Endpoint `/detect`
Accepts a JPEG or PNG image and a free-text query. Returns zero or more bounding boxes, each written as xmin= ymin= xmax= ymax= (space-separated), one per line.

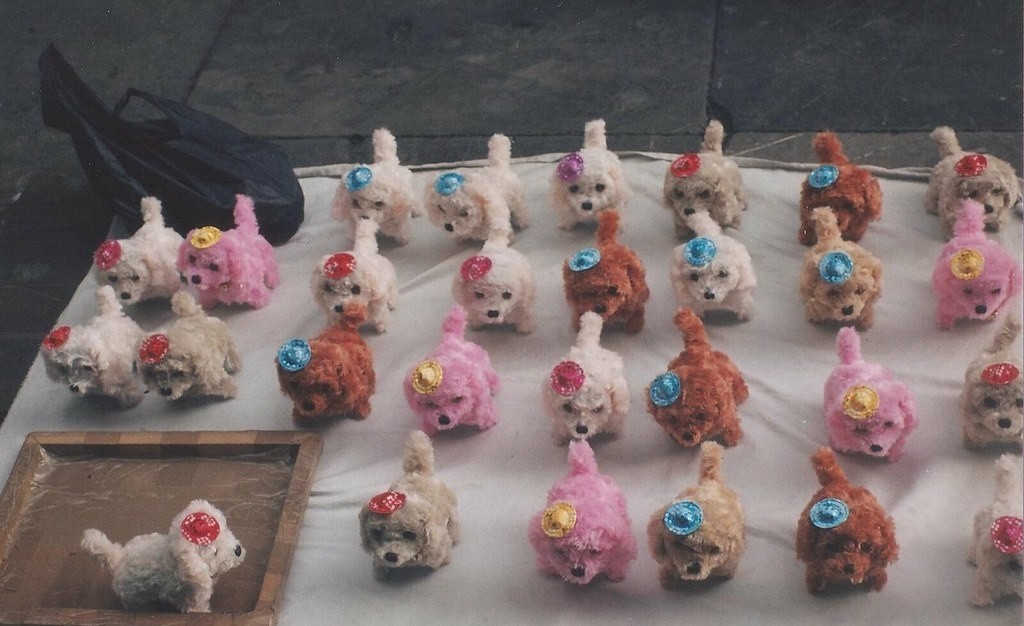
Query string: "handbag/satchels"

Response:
xmin=38 ymin=45 xmax=304 ymax=246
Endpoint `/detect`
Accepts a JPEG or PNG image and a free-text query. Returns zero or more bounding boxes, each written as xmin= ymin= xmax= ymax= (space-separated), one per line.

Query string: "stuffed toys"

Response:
xmin=358 ymin=432 xmax=460 ymax=579
xmin=40 ymin=285 xmax=148 ymax=409
xmin=968 ymin=453 xmax=1023 ymax=606
xmin=526 ymin=440 xmax=637 ymax=584
xmin=310 ymin=220 xmax=399 ymax=332
xmin=428 ymin=118 xmax=1024 ymax=333
xmin=404 ymin=306 xmax=501 ymax=436
xmin=647 ymin=440 xmax=746 ymax=591
xmin=824 ymin=326 xmax=916 ymax=457
xmin=93 ymin=196 xmax=185 ymax=306
xmin=645 ymin=307 xmax=748 ymax=446
xmin=795 ymin=449 xmax=900 ymax=592
xmin=177 ymin=194 xmax=280 ymax=310
xmin=960 ymin=312 xmax=1024 ymax=449
xmin=137 ymin=290 xmax=242 ymax=401
xmin=334 ymin=127 xmax=424 ymax=244
xmin=275 ymin=300 xmax=375 ymax=422
xmin=540 ymin=311 xmax=630 ymax=444
xmin=82 ymin=499 xmax=246 ymax=613
